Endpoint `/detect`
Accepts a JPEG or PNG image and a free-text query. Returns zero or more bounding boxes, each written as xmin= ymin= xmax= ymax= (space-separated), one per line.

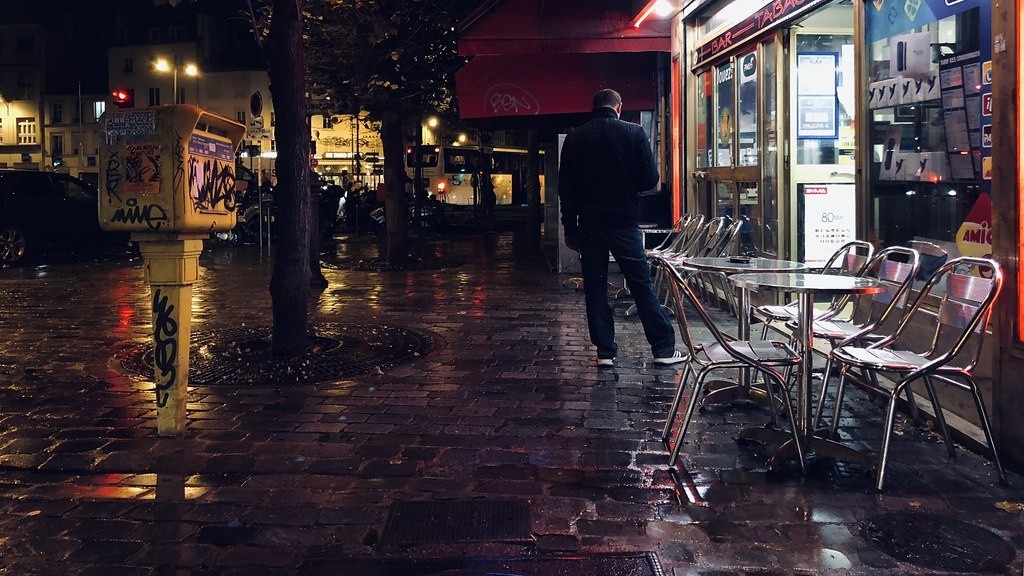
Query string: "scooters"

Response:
xmin=234 ymin=151 xmax=384 ymax=231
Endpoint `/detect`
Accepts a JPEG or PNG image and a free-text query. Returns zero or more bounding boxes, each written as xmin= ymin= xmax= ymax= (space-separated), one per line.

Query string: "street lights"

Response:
xmin=156 ymin=60 xmax=198 ymax=103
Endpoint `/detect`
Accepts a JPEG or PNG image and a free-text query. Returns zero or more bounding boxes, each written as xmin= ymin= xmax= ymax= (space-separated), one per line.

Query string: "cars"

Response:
xmin=0 ymin=169 xmax=98 ymax=229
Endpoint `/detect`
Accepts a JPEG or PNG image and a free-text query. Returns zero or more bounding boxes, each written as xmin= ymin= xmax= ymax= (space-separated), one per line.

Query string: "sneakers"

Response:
xmin=596 ymin=355 xmax=615 ymax=365
xmin=652 ymin=348 xmax=687 ymax=364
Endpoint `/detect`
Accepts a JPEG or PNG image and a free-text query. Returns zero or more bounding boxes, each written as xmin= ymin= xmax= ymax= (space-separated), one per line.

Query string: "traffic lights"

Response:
xmin=112 ymin=89 xmax=131 ymax=105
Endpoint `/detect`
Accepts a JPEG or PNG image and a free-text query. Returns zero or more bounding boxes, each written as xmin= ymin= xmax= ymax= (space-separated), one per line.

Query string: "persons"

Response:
xmin=363 ymin=183 xmax=369 ymax=194
xmin=556 ymin=89 xmax=690 ymax=367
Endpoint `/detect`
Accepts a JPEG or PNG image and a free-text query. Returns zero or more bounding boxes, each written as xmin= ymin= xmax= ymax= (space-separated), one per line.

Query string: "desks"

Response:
xmin=683 ymin=257 xmax=809 ymax=416
xmin=615 ymin=224 xmax=679 ymax=316
xmin=727 ymin=273 xmax=888 ymax=478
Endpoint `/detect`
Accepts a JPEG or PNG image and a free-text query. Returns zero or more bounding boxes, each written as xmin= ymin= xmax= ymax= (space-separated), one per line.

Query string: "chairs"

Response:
xmin=643 ymin=213 xmax=1008 ymax=490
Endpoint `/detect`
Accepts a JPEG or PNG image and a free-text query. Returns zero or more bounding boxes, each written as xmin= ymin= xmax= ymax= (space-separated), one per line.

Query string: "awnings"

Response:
xmin=455 ymin=0 xmax=672 ymax=118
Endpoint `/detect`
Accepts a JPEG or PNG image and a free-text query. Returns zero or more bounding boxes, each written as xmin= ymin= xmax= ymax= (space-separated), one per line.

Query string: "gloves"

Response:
xmin=565 ymin=236 xmax=578 ymax=250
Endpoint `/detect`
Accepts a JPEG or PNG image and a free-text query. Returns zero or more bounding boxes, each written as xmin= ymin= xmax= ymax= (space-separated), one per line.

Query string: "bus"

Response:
xmin=404 ymin=144 xmax=546 ymax=208
